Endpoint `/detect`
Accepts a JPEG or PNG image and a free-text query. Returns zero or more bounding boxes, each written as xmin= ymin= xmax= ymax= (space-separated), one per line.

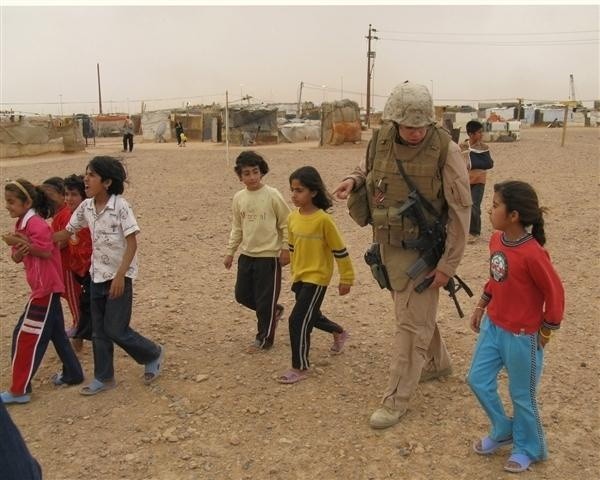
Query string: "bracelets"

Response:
xmin=474 ymin=306 xmax=486 ymax=312
xmin=538 ymin=327 xmax=554 ymax=339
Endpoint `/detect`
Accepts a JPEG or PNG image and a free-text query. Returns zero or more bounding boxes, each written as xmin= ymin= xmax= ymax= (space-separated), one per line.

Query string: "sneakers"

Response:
xmin=420 ymin=367 xmax=453 ymax=382
xmin=370 ymin=405 xmax=404 ymax=429
xmin=273 ymin=304 xmax=285 ymax=328
xmin=246 ymin=345 xmax=264 ymax=354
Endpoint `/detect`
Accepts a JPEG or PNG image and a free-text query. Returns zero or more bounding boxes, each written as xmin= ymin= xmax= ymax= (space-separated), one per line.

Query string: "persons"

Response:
xmin=276 ymin=165 xmax=355 ymax=385
xmin=178 ymin=131 xmax=186 ymax=147
xmin=51 ymin=156 xmax=165 ymax=396
xmin=1 ymin=177 xmax=84 ymax=402
xmin=464 ymin=180 xmax=566 ymax=473
xmin=330 ymin=81 xmax=473 ymax=429
xmin=458 ymin=120 xmax=494 ymax=244
xmin=43 ymin=175 xmax=92 ymax=337
xmin=120 ymin=114 xmax=135 ymax=153
xmin=174 ymin=118 xmax=184 ymax=145
xmin=222 ymin=149 xmax=292 ymax=349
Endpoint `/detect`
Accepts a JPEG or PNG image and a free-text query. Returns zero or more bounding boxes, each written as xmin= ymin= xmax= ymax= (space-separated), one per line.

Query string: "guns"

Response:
xmin=397 ymin=196 xmax=473 ymax=318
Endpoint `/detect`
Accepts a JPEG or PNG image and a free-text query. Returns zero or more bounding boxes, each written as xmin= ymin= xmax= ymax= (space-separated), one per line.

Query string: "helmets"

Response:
xmin=380 ymin=82 xmax=437 ymax=128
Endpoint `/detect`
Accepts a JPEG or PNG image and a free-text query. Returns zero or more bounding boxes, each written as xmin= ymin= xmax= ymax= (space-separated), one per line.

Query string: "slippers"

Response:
xmin=505 ymin=453 xmax=532 ymax=473
xmin=144 ymin=344 xmax=165 ymax=384
xmin=51 ymin=373 xmax=66 ymax=385
xmin=467 ymin=233 xmax=480 ymax=244
xmin=78 ymin=380 xmax=117 ymax=396
xmin=1 ymin=390 xmax=29 ymax=403
xmin=275 ymin=368 xmax=307 ymax=383
xmin=473 ymin=435 xmax=513 ymax=454
xmin=330 ymin=330 xmax=349 ymax=354
